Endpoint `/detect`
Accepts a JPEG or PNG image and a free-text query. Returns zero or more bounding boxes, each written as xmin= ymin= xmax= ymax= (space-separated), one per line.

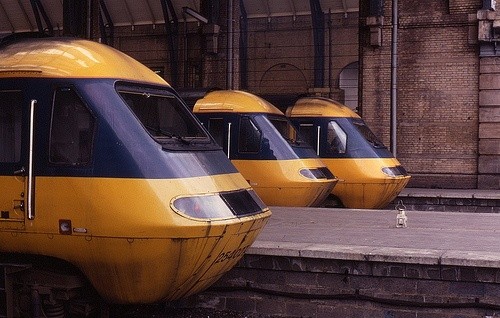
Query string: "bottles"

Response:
xmin=396 ymin=209 xmax=407 ymax=229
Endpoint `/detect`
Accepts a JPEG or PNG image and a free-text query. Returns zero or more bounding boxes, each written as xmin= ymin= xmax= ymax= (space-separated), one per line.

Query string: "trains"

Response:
xmin=258 ymin=93 xmax=413 ymax=210
xmin=177 ymin=88 xmax=337 ymax=208
xmin=0 ymin=36 xmax=274 ymax=318
